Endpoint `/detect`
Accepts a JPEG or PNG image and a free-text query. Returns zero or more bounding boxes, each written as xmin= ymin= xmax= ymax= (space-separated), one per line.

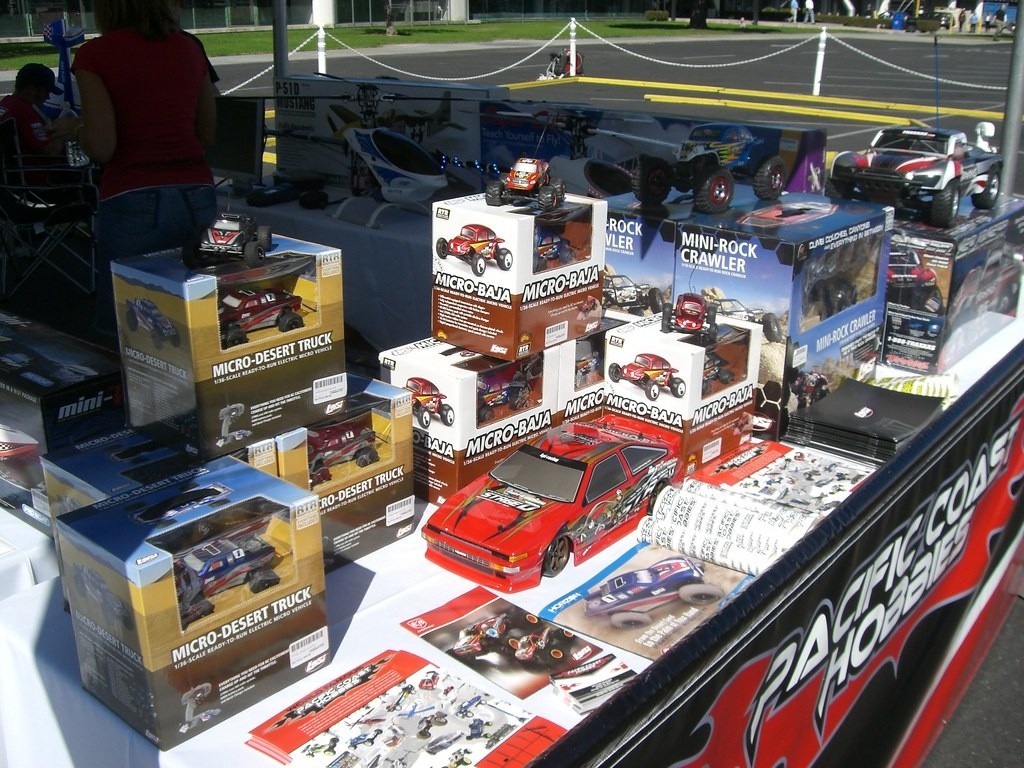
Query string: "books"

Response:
xmin=634 ymin=479 xmax=817 ymax=575
xmin=779 ymin=377 xmax=945 ymax=473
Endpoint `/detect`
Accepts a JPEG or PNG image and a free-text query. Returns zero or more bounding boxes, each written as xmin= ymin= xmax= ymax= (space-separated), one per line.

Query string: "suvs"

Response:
xmin=635 ymin=119 xmax=787 ymax=216
xmin=922 ymin=11 xmax=956 ymax=30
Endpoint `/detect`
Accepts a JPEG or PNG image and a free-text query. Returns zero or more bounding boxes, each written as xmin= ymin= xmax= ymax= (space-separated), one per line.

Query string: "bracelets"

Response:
xmin=73 ymin=122 xmax=88 ymax=139
xmin=49 ymin=123 xmax=57 ymax=132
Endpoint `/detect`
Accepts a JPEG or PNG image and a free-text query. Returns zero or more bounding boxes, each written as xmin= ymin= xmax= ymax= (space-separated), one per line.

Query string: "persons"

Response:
xmin=957 ymin=8 xmax=967 ymax=32
xmin=40 ymin=0 xmax=222 ymax=347
xmin=787 ymin=0 xmax=799 ymax=24
xmin=984 ymin=12 xmax=992 ymax=32
xmin=970 ymin=12 xmax=979 ymax=33
xmin=865 ymin=4 xmax=873 ymax=19
xmin=991 ymin=3 xmax=1007 ymax=42
xmin=804 ymin=0 xmax=815 ymax=24
xmin=0 ymin=63 xmax=99 ymax=247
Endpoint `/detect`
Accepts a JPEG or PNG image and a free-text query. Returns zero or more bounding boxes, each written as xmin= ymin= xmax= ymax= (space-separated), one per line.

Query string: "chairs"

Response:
xmin=0 ymin=116 xmax=101 ymax=300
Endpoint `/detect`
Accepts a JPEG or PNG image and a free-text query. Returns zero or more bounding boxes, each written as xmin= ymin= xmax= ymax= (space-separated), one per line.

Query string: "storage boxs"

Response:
xmin=0 ymin=74 xmax=1024 ymax=751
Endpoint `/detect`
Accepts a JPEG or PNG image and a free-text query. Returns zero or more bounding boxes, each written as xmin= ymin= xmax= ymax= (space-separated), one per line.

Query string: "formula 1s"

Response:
xmin=126 ymin=296 xmax=181 ymax=350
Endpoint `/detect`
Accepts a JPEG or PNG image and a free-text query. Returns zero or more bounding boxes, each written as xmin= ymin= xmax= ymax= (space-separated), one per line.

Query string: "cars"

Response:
xmin=438 ymin=223 xmax=516 ymax=274
xmin=830 ymin=127 xmax=1005 ymax=228
xmin=305 ymin=422 xmax=382 ymax=489
xmin=183 ymin=208 xmax=275 ymax=268
xmin=173 ymin=535 xmax=282 ymax=629
xmin=663 ymin=293 xmax=722 ymax=344
xmin=486 ymin=157 xmax=567 ymax=216
xmin=222 ymin=285 xmax=306 ymax=343
xmin=303 ymin=671 xmax=509 ymax=768
xmin=609 ymin=354 xmax=687 ymax=401
xmin=447 ymin=607 xmax=577 ymax=672
xmin=886 ymin=243 xmax=944 ymax=313
xmin=417 ymin=417 xmax=683 ymax=594
xmin=583 ymin=557 xmax=723 ymax=628
xmin=405 ymin=378 xmax=455 ymax=425
xmin=535 ymin=230 xmax=579 ymax=275
